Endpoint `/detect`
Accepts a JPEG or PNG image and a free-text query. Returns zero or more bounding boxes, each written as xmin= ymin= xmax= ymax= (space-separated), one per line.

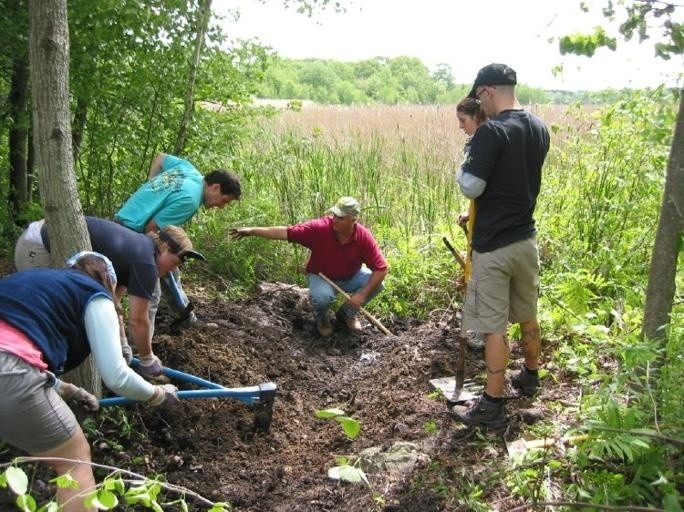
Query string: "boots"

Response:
xmin=453 ymin=391 xmax=506 ymax=424
xmin=511 ymin=366 xmax=539 ymax=395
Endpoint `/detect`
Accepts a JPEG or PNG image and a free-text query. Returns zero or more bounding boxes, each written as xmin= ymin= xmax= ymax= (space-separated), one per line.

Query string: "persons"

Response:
xmin=13 ymin=216 xmax=192 ymax=377
xmin=452 ymin=63 xmax=550 ymax=426
xmin=0 ymin=251 xmax=181 ymax=512
xmin=114 ymin=153 xmax=241 ymax=332
xmin=229 ymin=196 xmax=390 ymax=337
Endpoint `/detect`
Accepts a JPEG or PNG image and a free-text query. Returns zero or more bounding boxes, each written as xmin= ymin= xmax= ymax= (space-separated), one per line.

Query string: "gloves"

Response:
xmin=61 ymin=383 xmax=99 ymax=412
xmin=121 ymin=337 xmax=180 ymax=407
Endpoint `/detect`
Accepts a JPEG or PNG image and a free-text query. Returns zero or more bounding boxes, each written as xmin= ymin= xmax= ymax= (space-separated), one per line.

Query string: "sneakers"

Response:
xmin=317 ymin=308 xmax=362 ymax=336
xmin=172 ymin=312 xmax=218 ymax=337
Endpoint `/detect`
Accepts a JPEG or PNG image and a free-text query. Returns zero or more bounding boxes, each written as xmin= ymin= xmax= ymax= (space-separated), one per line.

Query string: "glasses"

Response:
xmin=475 ymin=84 xmax=496 ymax=105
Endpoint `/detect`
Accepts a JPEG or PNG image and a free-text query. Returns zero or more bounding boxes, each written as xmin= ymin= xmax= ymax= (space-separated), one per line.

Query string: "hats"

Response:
xmin=325 ymin=196 xmax=360 ymax=217
xmin=467 ymin=64 xmax=516 ymax=98
